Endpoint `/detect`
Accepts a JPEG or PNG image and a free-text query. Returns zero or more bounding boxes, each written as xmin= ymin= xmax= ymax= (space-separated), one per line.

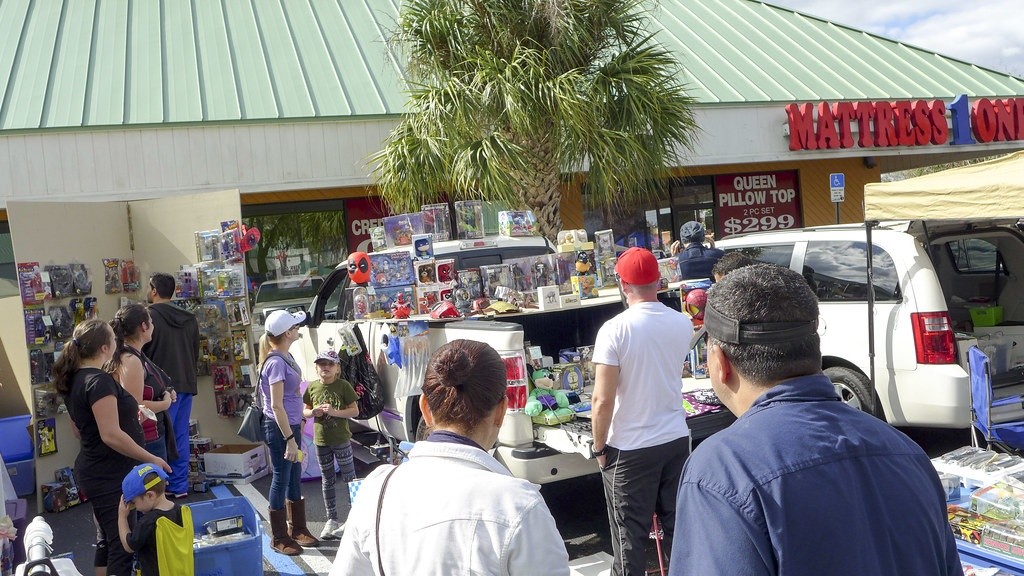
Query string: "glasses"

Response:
xmin=615 ymin=272 xmax=620 ymax=281
xmin=281 ymin=323 xmax=300 ymax=335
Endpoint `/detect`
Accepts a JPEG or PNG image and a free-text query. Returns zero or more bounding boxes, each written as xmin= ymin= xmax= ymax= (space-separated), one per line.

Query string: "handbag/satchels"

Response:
xmin=237 ymin=403 xmax=263 ymax=442
xmin=338 ymin=323 xmax=385 ymax=420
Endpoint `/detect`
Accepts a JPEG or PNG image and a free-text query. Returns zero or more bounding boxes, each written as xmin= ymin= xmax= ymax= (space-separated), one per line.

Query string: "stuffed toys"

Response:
xmin=416 ymin=239 xmax=431 ymax=256
xmin=524 ymin=369 xmax=573 ymax=416
xmin=420 ymin=266 xmax=433 ymax=283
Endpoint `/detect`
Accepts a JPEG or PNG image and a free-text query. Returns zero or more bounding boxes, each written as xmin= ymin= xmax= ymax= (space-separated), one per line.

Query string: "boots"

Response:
xmin=286 ymin=495 xmax=319 ymax=547
xmin=268 ymin=504 xmax=304 ymax=554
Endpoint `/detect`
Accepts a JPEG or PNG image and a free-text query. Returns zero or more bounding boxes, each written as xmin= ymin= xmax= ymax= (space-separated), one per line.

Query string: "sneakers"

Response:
xmin=332 ymin=523 xmax=346 ymax=538
xmin=320 ymin=519 xmax=339 ymax=539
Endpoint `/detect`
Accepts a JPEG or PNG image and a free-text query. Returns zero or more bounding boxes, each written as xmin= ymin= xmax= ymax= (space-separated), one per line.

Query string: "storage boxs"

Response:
xmin=0 ymin=415 xmax=33 ymax=461
xmin=528 ymin=346 xmax=542 ymax=360
xmin=4 ymin=458 xmax=36 ymax=543
xmin=186 ymin=366 xmax=265 ymax=476
xmin=952 ymin=306 xmax=1024 ymax=375
xmin=181 ymin=495 xmax=263 ymax=576
xmin=541 ymin=356 xmax=554 ymax=368
xmin=363 ymin=200 xmax=618 ymax=320
xmin=42 ymin=466 xmax=84 ymax=513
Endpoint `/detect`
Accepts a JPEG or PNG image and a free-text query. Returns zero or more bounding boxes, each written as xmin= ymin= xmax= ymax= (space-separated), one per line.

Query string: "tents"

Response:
xmin=864 ymin=150 xmax=1024 ymax=419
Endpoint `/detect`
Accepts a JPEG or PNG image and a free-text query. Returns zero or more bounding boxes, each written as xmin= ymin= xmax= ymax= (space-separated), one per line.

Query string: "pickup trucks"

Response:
xmin=251 ymin=275 xmax=327 ymax=355
xmin=288 ymin=233 xmax=737 ymax=522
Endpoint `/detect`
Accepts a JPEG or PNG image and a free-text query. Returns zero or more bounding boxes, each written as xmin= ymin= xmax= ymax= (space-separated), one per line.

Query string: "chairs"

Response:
xmin=964 ymin=346 xmax=1024 ymax=456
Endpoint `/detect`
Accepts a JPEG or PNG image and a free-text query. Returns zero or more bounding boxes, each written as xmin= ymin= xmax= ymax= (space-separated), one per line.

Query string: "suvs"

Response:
xmin=703 ymin=222 xmax=1024 ymax=429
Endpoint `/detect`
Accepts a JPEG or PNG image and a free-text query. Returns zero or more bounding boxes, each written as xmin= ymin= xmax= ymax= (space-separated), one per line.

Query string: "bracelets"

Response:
xmin=592 ymin=442 xmax=608 ymax=456
xmin=283 ymin=433 xmax=295 ymax=441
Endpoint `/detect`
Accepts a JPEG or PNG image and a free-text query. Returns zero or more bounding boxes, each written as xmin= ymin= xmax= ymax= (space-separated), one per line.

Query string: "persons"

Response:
xmin=713 ymin=252 xmax=753 ymax=281
xmin=671 ymin=221 xmax=725 ymax=283
xmin=353 ymin=258 xmax=414 ymax=320
xmin=103 ymin=305 xmax=176 ymax=519
xmin=591 ymin=248 xmax=694 ymax=576
xmin=258 ymin=310 xmax=319 ymax=553
xmin=53 ymin=319 xmax=172 ymax=576
xmin=118 ymin=463 xmax=194 ymax=576
xmin=303 ymin=351 xmax=359 ymax=540
xmin=142 ymin=272 xmax=199 ymax=498
xmin=669 ymin=262 xmax=964 ymax=576
xmin=329 ymin=339 xmax=571 ymax=576
xmin=513 ymin=255 xmax=575 ymax=291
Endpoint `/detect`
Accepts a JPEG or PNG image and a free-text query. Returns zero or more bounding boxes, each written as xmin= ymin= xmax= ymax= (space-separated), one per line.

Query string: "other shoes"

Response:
xmin=164 ymin=479 xmax=169 ymax=486
xmin=165 ymin=490 xmax=188 ymax=499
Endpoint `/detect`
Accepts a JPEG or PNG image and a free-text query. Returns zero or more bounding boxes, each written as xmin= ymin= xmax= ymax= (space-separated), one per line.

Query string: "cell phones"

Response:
xmin=297 ymin=450 xmax=303 ymax=462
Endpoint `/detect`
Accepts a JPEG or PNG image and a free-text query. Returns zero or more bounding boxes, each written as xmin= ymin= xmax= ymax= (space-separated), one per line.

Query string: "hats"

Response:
xmin=679 ymin=221 xmax=705 ymax=242
xmin=122 ymin=463 xmax=169 ymax=510
xmin=314 ymin=351 xmax=341 ymax=364
xmin=265 ymin=309 xmax=306 ymax=337
xmin=690 ymin=299 xmax=819 ymax=350
xmin=615 ymin=247 xmax=658 ymax=284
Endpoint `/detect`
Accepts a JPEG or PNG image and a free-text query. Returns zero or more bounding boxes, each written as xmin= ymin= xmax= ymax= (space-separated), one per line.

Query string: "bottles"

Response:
xmin=204 ymin=479 xmax=226 ymax=487
